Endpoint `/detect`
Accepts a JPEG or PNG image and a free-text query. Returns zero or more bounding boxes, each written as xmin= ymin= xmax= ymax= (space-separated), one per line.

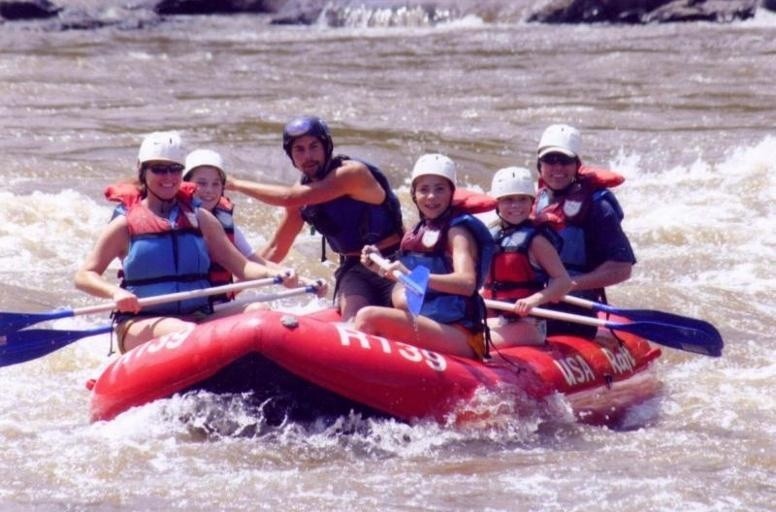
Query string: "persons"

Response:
xmin=527 ymin=124 xmax=638 ymax=340
xmin=474 ymin=165 xmax=576 ymax=351
xmin=106 ymin=147 xmax=332 ymax=305
xmin=217 ymin=112 xmax=411 ymax=322
xmin=353 ymin=150 xmax=487 ymax=362
xmin=70 ymin=127 xmax=302 ymax=356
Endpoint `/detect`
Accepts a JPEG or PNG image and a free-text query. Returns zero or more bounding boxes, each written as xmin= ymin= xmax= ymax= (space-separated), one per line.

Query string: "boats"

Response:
xmin=84 ymin=295 xmax=664 ymax=450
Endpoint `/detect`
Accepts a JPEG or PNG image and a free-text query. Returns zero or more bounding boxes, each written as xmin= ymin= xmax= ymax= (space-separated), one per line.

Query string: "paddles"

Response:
xmin=0 ymin=287 xmax=314 ymax=368
xmin=1 ymin=272 xmax=288 ymax=339
xmin=558 ymin=295 xmax=725 ymax=350
xmin=370 ymin=251 xmax=430 ymax=317
xmin=485 ymin=321 xmax=724 ymax=358
